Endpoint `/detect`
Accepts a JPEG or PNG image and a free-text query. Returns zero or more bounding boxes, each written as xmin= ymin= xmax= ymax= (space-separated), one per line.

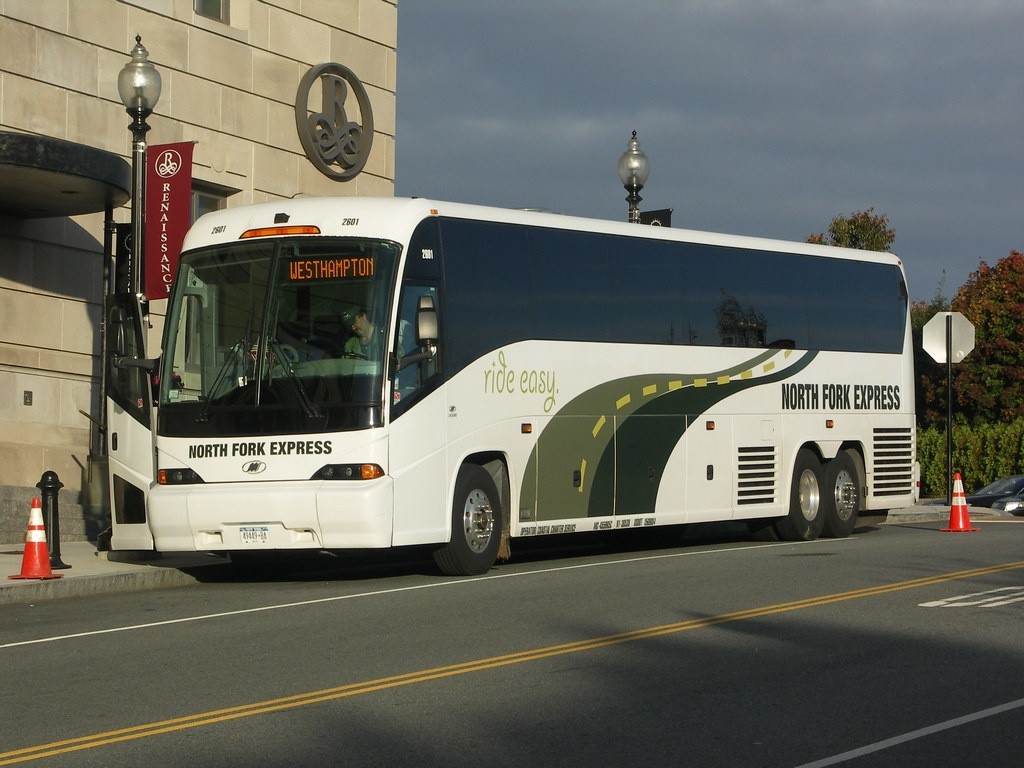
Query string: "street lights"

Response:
xmin=615 ymin=126 xmax=654 ymax=223
xmin=115 ymin=32 xmax=164 ymax=416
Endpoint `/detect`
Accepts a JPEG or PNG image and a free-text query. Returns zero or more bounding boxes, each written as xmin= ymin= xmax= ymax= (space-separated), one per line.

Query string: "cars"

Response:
xmin=929 ymin=473 xmax=1024 ymax=516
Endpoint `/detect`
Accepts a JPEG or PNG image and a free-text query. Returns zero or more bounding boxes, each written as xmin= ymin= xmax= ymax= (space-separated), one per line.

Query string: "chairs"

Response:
xmin=314 ymin=314 xmax=345 ymax=343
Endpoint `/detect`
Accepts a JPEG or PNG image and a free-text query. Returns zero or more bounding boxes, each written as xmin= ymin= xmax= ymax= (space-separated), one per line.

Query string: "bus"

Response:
xmin=98 ymin=193 xmax=922 ymax=578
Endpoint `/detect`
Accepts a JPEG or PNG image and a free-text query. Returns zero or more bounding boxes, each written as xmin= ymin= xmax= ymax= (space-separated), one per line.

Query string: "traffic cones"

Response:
xmin=939 ymin=472 xmax=983 ymax=532
xmin=7 ymin=498 xmax=68 ymax=580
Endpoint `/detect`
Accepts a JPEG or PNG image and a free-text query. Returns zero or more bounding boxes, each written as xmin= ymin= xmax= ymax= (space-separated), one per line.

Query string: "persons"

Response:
xmin=339 ymin=304 xmax=405 ymax=381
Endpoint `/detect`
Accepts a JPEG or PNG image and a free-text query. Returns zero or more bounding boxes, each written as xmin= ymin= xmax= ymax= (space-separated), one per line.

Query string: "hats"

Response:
xmin=339 ymin=302 xmax=363 ymax=327
